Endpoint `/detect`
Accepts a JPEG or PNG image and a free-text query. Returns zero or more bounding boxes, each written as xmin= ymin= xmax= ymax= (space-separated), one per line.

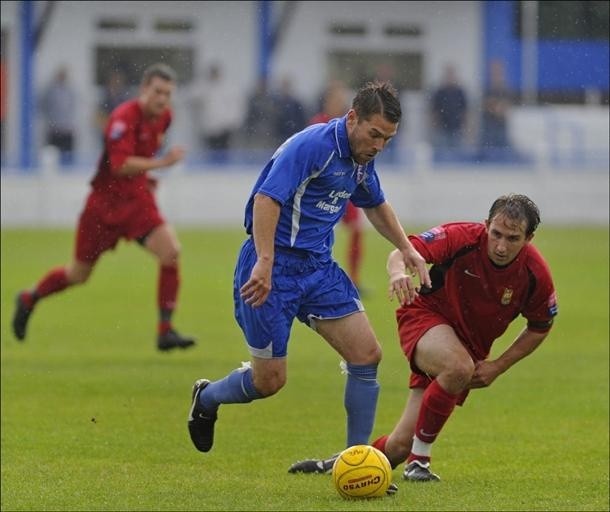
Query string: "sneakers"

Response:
xmin=187 ymin=378 xmax=219 ymax=453
xmin=13 ymin=292 xmax=32 ymax=341
xmin=289 ymin=455 xmax=442 ymax=498
xmin=158 ymin=328 xmax=195 ymax=351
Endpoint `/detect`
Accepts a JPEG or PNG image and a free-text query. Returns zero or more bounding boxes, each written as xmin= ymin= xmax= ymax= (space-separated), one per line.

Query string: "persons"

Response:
xmin=187 ymin=82 xmax=433 ymax=496
xmin=243 ymin=79 xmax=345 ymax=158
xmin=37 ymin=60 xmax=128 ymax=163
xmin=12 ymin=63 xmax=196 ymax=349
xmin=430 ymin=63 xmax=512 ymax=145
xmin=288 ymin=194 xmax=558 ymax=482
xmin=306 ymin=82 xmax=363 ymax=283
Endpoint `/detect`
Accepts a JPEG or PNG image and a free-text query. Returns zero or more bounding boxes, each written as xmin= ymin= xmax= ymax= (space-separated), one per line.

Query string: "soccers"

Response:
xmin=333 ymin=446 xmax=393 ymax=502
